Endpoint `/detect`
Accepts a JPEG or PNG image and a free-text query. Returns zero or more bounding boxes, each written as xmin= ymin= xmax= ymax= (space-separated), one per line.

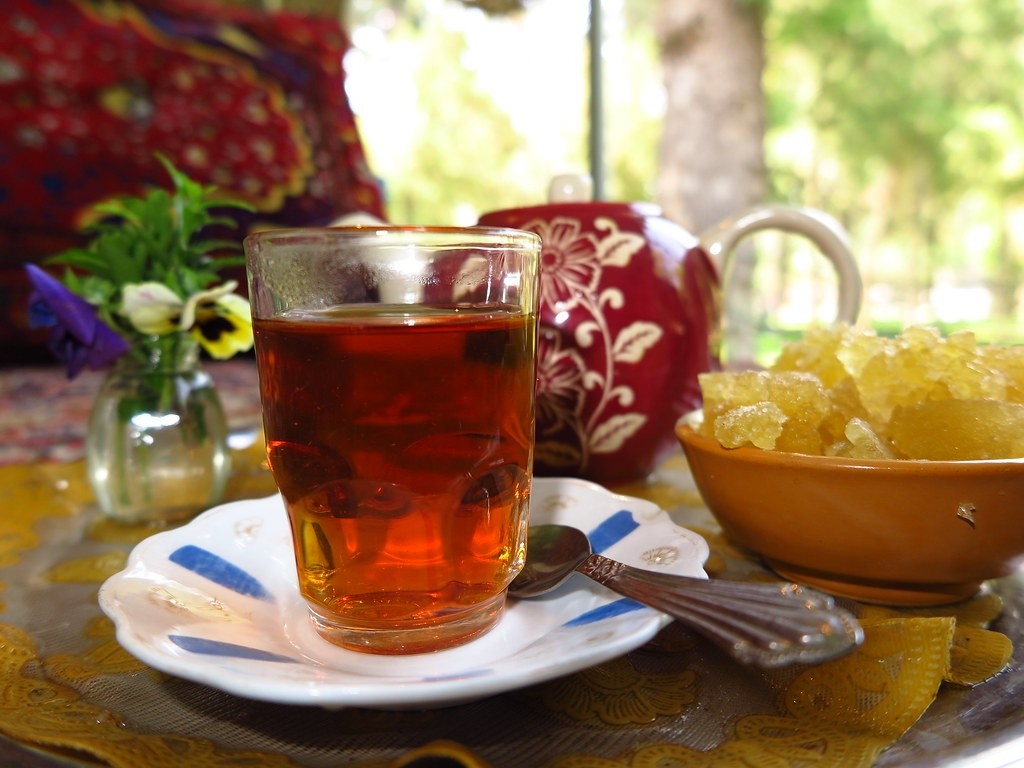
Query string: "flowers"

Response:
xmin=60 ymin=153 xmax=253 ymax=359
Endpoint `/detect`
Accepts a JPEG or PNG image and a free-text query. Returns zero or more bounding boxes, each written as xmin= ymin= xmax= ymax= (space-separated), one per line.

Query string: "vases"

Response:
xmin=86 ymin=332 xmax=230 ymax=518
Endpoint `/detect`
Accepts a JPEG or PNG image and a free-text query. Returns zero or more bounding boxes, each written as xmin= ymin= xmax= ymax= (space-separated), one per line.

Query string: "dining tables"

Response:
xmin=0 ymin=330 xmax=1024 ymax=768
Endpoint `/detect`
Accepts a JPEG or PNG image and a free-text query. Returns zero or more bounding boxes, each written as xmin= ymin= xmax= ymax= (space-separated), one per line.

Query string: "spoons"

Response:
xmin=506 ymin=524 xmax=863 ymax=666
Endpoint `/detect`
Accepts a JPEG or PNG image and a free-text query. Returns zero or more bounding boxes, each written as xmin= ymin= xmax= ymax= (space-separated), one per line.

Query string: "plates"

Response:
xmin=98 ymin=478 xmax=710 ymax=708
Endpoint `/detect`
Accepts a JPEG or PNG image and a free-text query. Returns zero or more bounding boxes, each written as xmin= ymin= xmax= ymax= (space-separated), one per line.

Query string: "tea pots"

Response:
xmin=327 ymin=174 xmax=861 ymax=489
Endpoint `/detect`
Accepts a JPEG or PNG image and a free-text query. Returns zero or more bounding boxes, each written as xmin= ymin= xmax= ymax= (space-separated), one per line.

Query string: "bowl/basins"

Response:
xmin=673 ymin=406 xmax=1023 ymax=608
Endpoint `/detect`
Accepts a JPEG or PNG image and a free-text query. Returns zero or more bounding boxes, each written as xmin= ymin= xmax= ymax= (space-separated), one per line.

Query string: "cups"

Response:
xmin=243 ymin=225 xmax=543 ymax=654
